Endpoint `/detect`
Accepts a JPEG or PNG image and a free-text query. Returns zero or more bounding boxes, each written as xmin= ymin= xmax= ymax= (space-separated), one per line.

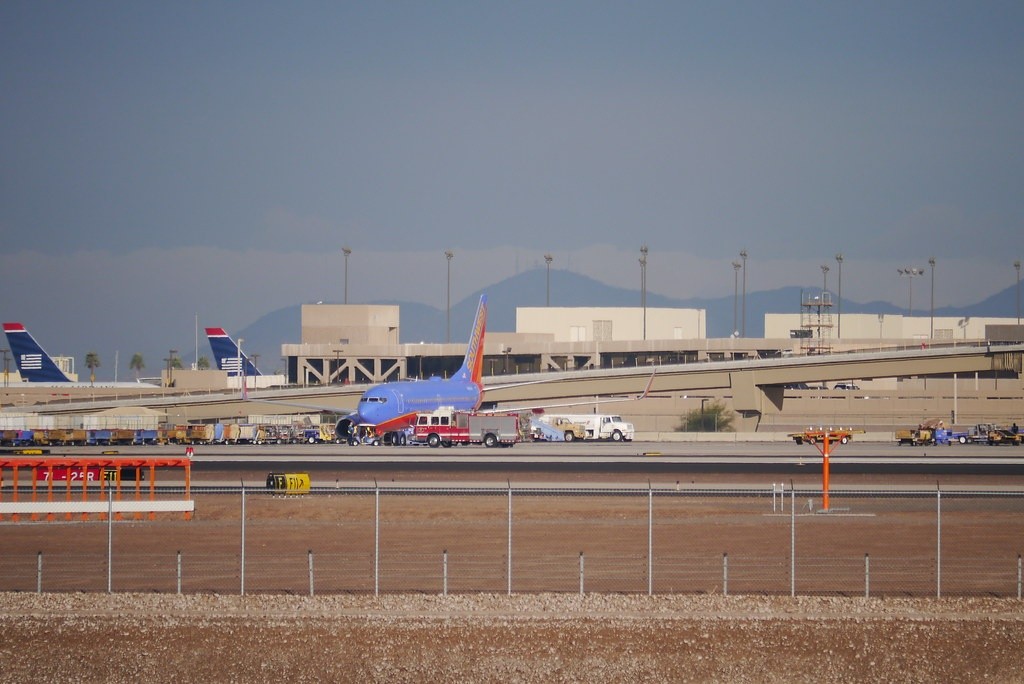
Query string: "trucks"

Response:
xmin=544 ymin=417 xmax=586 ymax=442
xmin=543 ymin=415 xmax=634 ymax=442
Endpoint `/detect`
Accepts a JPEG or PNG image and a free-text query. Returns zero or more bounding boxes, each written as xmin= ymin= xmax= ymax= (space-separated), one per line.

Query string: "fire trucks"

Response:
xmin=407 ymin=405 xmax=532 ymax=450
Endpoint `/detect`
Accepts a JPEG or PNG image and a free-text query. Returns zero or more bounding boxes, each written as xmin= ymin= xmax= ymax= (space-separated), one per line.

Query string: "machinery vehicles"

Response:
xmin=0 ymin=423 xmax=382 ymax=448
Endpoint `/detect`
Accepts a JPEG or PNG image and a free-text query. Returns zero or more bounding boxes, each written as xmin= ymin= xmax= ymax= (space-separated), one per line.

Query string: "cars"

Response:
xmin=783 ymin=381 xmax=863 ymax=400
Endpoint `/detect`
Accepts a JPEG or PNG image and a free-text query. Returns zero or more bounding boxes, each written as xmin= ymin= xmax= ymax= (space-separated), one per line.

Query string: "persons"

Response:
xmin=348 ymin=422 xmax=354 ymax=446
xmin=1012 ymin=422 xmax=1018 ymax=434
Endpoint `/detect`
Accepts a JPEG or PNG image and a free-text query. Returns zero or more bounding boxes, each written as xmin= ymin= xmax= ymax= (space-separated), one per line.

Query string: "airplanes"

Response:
xmin=239 ymin=294 xmax=659 ymax=447
xmin=205 ymin=326 xmax=264 ymax=378
xmin=0 ymin=320 xmax=163 ymax=389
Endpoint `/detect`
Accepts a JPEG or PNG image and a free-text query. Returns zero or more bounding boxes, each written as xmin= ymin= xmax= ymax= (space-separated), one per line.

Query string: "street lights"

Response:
xmin=928 ymin=255 xmax=937 ymax=340
xmin=500 ymin=346 xmax=512 ymax=375
xmin=897 ymin=267 xmax=925 ymax=319
xmin=876 ymin=312 xmax=885 ymax=353
xmin=340 ymin=245 xmax=355 ymax=305
xmin=959 ymin=315 xmax=969 ymax=340
xmin=835 ymin=252 xmax=845 ymax=339
xmin=731 ymin=260 xmax=743 ymax=337
xmin=738 ymin=248 xmax=749 ymax=338
xmin=543 ymin=254 xmax=554 ymax=307
xmin=167 ymin=339 xmax=344 ymax=392
xmin=639 ymin=247 xmax=649 ymax=340
xmin=444 ymin=250 xmax=455 ymax=342
xmin=1013 ymin=260 xmax=1022 ymax=326
xmin=820 ymin=263 xmax=829 ymax=293
